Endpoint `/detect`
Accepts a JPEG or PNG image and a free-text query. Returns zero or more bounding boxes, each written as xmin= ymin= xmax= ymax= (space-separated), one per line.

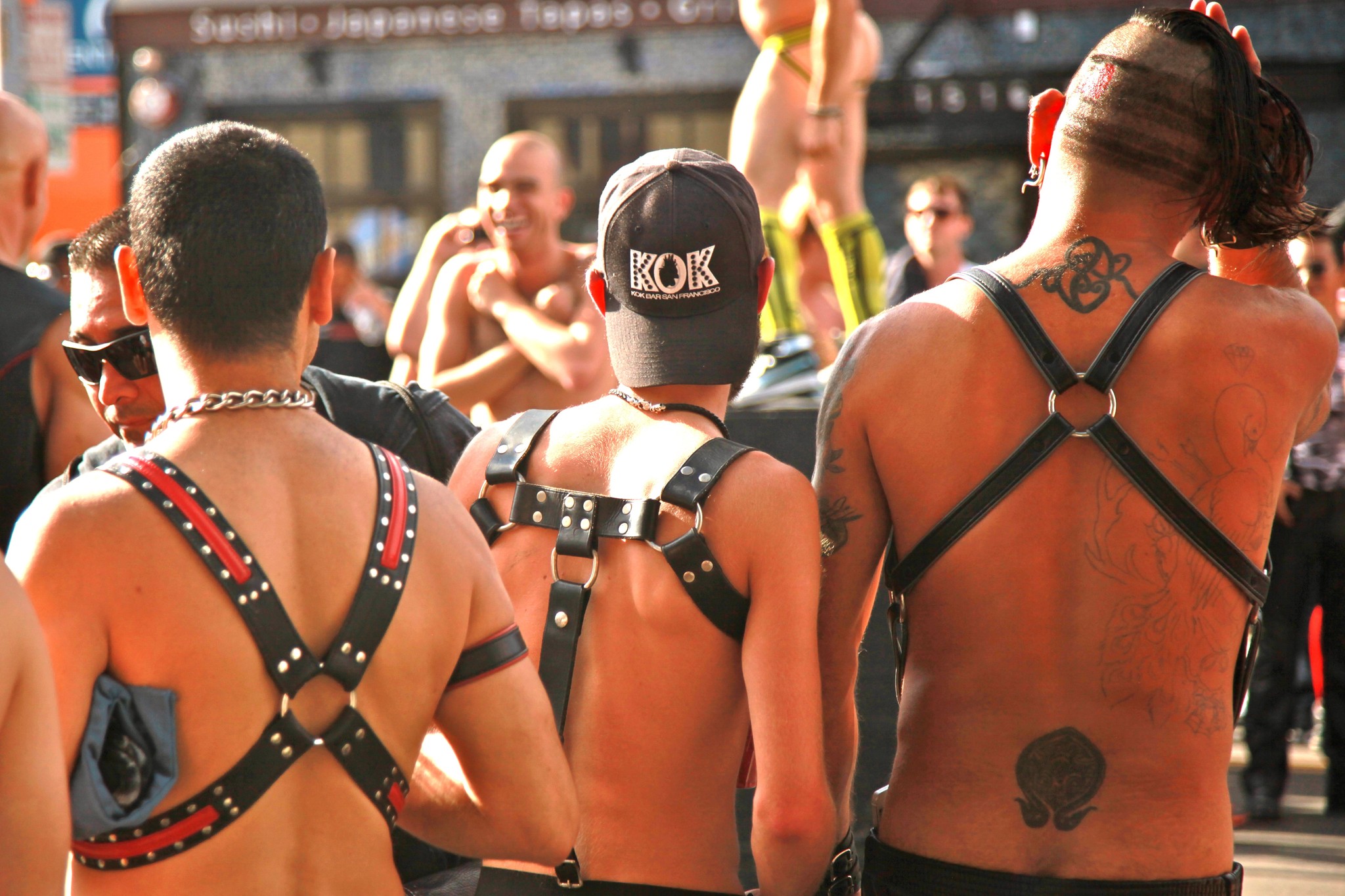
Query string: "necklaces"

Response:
xmin=145 ymin=389 xmax=315 ymax=443
xmin=608 ymin=389 xmax=731 ymax=441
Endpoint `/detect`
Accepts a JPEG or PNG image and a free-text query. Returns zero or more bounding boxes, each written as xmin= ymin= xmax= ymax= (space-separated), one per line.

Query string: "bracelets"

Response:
xmin=823 ymin=830 xmax=861 ymax=896
xmin=1201 ymin=223 xmax=1268 ymax=250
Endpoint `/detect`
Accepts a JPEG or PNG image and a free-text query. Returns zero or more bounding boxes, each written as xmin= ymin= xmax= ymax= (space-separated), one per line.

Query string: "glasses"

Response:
xmin=60 ymin=328 xmax=154 ymax=381
xmin=1294 ymin=260 xmax=1341 ymax=276
xmin=903 ymin=207 xmax=967 ymax=220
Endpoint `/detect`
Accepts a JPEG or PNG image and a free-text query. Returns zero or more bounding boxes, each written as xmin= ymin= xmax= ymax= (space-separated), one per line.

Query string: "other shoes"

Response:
xmin=1250 ymin=781 xmax=1278 ymax=825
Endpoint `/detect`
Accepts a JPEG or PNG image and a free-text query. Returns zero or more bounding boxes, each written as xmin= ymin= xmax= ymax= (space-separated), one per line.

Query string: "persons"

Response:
xmin=4 ymin=117 xmax=579 ymax=896
xmin=0 ymin=556 xmax=72 ymax=896
xmin=414 ymin=131 xmax=616 ymax=432
xmin=61 ymin=214 xmax=484 ymax=482
xmin=448 ymin=143 xmax=836 ymax=896
xmin=725 ymin=0 xmax=985 ymax=370
xmin=1172 ymin=204 xmax=1345 ymax=832
xmin=0 ymin=90 xmax=113 ymax=553
xmin=807 ymin=0 xmax=1340 ymax=896
xmin=321 ymin=235 xmax=394 ymax=349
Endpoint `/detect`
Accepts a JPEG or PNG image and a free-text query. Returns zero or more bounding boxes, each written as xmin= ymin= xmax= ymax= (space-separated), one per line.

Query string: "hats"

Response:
xmin=595 ymin=148 xmax=764 ymax=389
